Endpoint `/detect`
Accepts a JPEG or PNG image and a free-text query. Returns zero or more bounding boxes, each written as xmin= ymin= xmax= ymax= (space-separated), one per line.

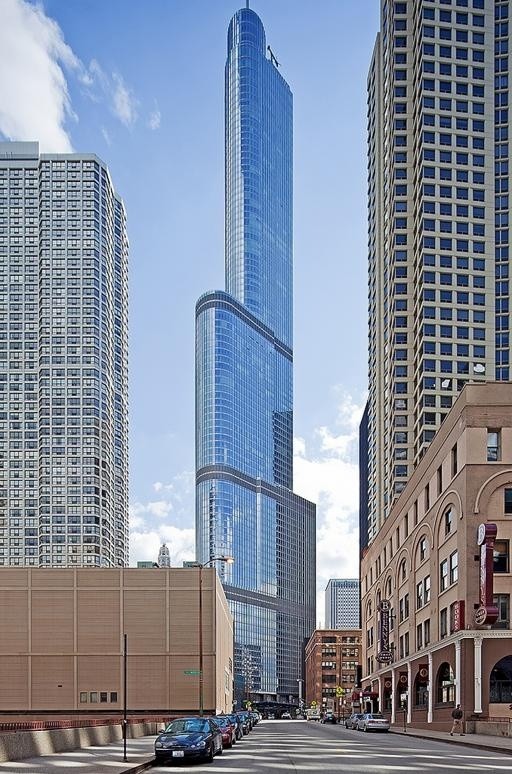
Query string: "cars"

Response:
xmin=282 ymin=713 xmax=290 ymax=719
xmin=268 ymin=715 xmax=275 ymax=719
xmin=344 ymin=713 xmax=364 ymax=729
xmin=210 ymin=711 xmax=261 ymax=749
xmin=356 ymin=713 xmax=391 ymax=733
xmin=154 ymin=718 xmax=222 ymax=764
xmin=320 ymin=712 xmax=337 ymax=724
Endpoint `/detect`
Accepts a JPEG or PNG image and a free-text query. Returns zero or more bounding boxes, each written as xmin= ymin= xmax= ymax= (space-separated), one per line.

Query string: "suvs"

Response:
xmin=306 ymin=708 xmax=321 ymax=722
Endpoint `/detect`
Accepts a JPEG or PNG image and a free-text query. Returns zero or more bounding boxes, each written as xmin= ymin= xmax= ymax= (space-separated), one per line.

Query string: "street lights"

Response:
xmin=197 ymin=556 xmax=235 ymax=718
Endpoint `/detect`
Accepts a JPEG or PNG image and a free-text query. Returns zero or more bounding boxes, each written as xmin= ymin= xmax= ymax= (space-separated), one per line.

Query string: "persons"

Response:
xmin=450 ymin=704 xmax=464 ymax=736
xmin=402 ymin=697 xmax=408 ymax=722
